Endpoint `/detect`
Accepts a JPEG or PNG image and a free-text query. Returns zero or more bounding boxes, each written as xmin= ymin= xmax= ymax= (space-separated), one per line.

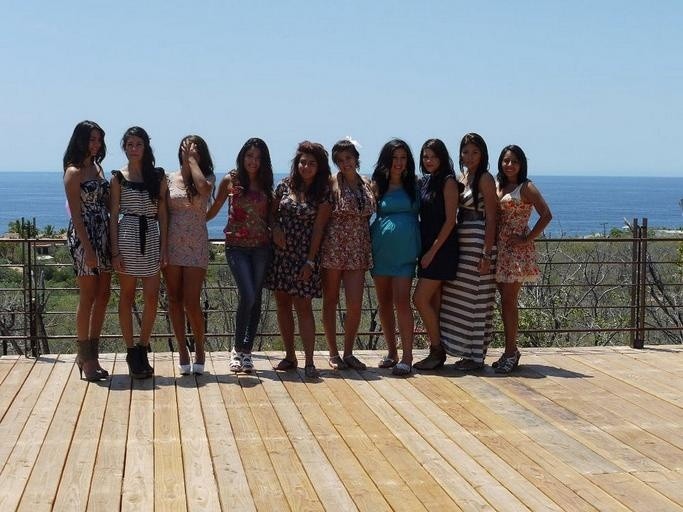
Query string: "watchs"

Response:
xmin=306 ymin=259 xmax=320 ymax=272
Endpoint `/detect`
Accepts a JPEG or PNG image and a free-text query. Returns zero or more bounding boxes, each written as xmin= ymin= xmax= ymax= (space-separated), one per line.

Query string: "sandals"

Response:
xmin=414 ymin=345 xmax=446 ymax=370
xmin=241 ymin=353 xmax=253 ymax=372
xmin=495 ymin=350 xmax=521 ymax=374
xmin=228 ymin=348 xmax=242 ymax=372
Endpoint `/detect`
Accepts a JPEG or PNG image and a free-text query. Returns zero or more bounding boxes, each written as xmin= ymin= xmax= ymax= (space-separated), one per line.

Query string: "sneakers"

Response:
xmin=329 ymin=355 xmax=348 ymax=370
xmin=343 ymin=354 xmax=366 ymax=371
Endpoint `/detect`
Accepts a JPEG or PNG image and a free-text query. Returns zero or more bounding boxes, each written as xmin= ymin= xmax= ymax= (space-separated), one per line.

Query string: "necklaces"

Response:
xmin=342 ymin=174 xmax=365 ymax=212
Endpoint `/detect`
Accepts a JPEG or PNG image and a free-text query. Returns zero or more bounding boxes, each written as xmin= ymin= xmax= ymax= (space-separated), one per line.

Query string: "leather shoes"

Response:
xmin=492 ymin=353 xmax=504 ymax=368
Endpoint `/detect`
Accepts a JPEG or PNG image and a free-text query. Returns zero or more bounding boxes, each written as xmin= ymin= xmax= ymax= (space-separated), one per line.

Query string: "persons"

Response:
xmin=271 ymin=141 xmax=335 ymax=378
xmin=109 ymin=126 xmax=167 ymax=379
xmin=206 ymin=138 xmax=276 ymax=372
xmin=440 ymin=133 xmax=501 ymax=373
xmin=370 ymin=140 xmax=424 ymax=376
xmin=320 ymin=140 xmax=374 ymax=371
xmin=412 ymin=139 xmax=466 ymax=372
xmin=492 ymin=144 xmax=552 ymax=375
xmin=63 ymin=120 xmax=115 ymax=382
xmin=166 ymin=134 xmax=216 ymax=375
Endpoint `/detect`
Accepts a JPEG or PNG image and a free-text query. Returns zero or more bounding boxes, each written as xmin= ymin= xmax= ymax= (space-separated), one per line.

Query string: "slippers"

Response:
xmin=378 ymin=356 xmax=396 ymax=368
xmin=304 ymin=364 xmax=320 ymax=378
xmin=272 ymin=359 xmax=298 ymax=370
xmin=392 ymin=363 xmax=411 ymax=375
xmin=454 ymin=359 xmax=486 ymax=371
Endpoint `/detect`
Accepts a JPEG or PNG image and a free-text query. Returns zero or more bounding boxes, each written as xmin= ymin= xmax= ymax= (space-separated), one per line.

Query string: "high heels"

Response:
xmin=126 ymin=346 xmax=146 ymax=379
xmin=75 ymin=340 xmax=100 ymax=381
xmin=178 ymin=347 xmax=191 ymax=376
xmin=136 ymin=343 xmax=153 ymax=378
xmin=191 ymin=354 xmax=205 ymax=375
xmin=90 ymin=338 xmax=109 ymax=379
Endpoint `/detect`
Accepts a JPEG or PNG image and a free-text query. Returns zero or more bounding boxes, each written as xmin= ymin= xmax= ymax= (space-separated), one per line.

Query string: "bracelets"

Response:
xmin=111 ymin=252 xmax=121 ymax=258
xmin=481 ymin=251 xmax=494 ymax=261
